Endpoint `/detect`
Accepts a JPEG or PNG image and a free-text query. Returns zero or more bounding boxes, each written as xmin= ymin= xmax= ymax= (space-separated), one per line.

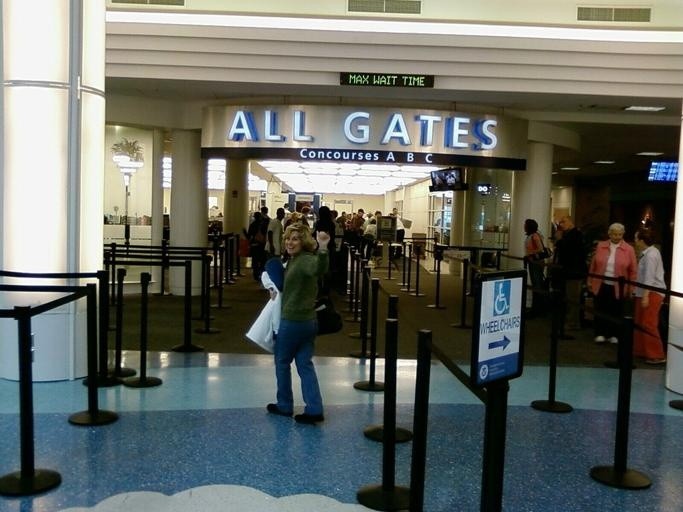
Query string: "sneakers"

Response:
xmin=644 ymin=356 xmax=666 ymax=365
xmin=264 ymin=401 xmax=294 ymax=417
xmin=607 ymin=335 xmax=618 ymax=344
xmin=594 ymin=333 xmax=606 ymax=343
xmin=293 ymin=411 xmax=325 ymax=424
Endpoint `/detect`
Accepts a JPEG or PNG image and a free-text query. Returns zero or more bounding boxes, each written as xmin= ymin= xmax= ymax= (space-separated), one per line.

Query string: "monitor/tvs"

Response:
xmin=647 ymin=160 xmax=679 ymax=184
xmin=430 ymin=167 xmax=463 ymax=187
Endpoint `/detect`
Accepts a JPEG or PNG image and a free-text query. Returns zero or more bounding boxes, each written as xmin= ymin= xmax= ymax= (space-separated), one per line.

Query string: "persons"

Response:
xmin=266 ymin=222 xmax=332 ymax=423
xmin=522 ymin=218 xmax=545 ymax=320
xmin=245 ymin=203 xmax=407 ymax=282
xmin=587 ymin=222 xmax=638 ymax=343
xmin=548 ymin=215 xmax=588 ymax=341
xmin=633 ymin=228 xmax=667 ymax=364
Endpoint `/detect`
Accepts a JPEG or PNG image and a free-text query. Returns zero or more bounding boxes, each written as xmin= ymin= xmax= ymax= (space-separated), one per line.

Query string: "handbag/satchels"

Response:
xmin=532 ymin=247 xmax=553 ymax=261
xmin=315 ymin=298 xmax=343 ymax=336
